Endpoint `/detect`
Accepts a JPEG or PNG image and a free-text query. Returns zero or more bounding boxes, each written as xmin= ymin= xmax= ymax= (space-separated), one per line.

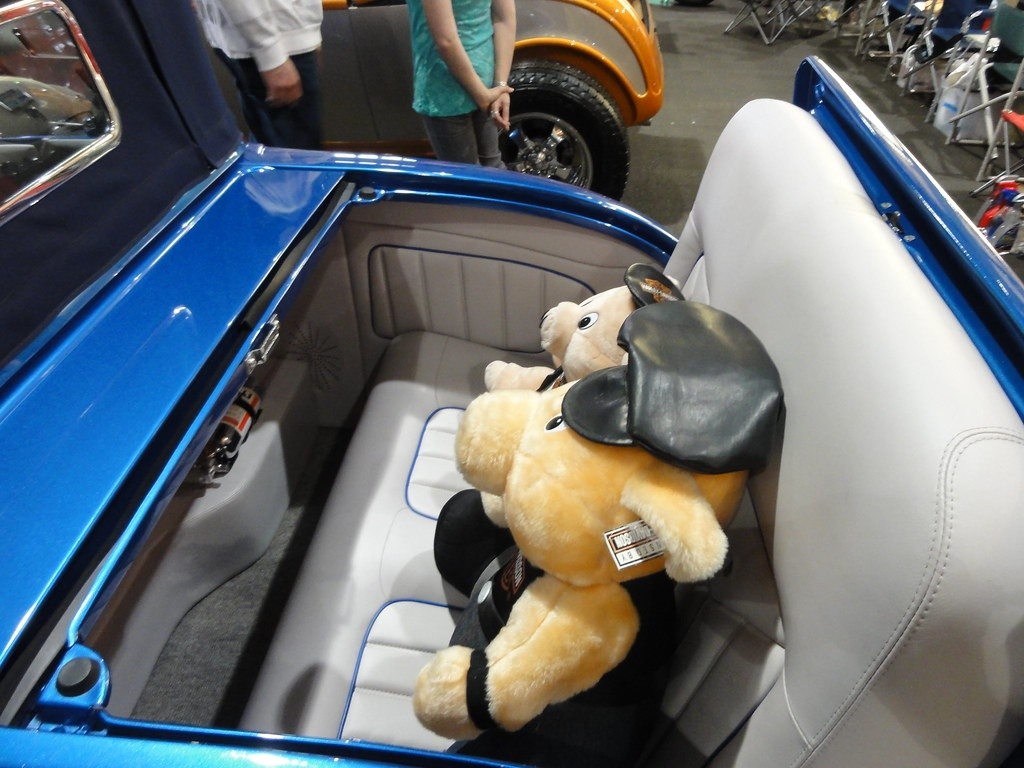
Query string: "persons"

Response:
xmin=196 ymin=0 xmax=324 ymax=150
xmin=405 ymin=0 xmax=517 ymax=170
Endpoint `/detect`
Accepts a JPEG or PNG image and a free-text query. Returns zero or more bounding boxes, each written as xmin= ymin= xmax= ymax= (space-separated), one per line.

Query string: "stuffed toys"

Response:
xmin=413 ymin=262 xmax=783 ymax=768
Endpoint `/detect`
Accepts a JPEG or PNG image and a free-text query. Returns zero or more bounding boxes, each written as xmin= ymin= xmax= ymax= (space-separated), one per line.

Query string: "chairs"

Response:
xmin=723 ymin=0 xmax=1024 ymax=195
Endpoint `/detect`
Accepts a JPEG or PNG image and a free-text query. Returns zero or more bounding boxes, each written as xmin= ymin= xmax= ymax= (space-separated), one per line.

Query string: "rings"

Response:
xmin=491 ymin=111 xmax=498 ymax=117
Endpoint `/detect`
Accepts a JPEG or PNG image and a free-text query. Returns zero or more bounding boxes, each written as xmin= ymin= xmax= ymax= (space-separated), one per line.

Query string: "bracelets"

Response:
xmin=492 ymin=81 xmax=506 ymax=87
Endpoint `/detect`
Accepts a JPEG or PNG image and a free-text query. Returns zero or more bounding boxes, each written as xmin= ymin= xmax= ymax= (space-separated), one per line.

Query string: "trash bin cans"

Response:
xmin=938 ymin=72 xmax=1002 ymax=141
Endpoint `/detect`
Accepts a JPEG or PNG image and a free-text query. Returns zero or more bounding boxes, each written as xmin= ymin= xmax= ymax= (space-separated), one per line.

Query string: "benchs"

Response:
xmin=238 ymin=98 xmax=1024 ymax=767
